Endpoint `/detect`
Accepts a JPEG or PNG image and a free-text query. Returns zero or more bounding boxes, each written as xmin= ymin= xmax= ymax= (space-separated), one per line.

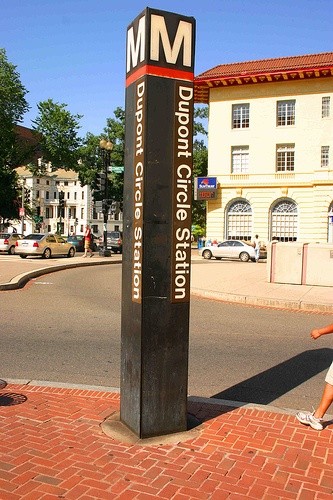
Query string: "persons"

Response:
xmin=70 ymin=231 xmax=74 ymax=235
xmin=55 ymin=230 xmax=59 ymax=234
xmin=82 ymin=224 xmax=94 ymax=258
xmin=255 ymin=234 xmax=261 ymax=263
xmin=296 ymin=323 xmax=333 ymax=430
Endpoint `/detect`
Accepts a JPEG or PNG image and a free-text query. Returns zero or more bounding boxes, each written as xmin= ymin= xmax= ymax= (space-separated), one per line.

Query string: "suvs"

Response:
xmin=99 ymin=230 xmax=123 ymax=254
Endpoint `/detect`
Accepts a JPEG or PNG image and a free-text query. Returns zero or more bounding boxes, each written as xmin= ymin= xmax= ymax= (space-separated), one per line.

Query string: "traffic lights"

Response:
xmin=59 ymin=199 xmax=64 ymax=207
xmin=21 ymin=187 xmax=31 ymax=204
xmin=36 ymin=206 xmax=40 ymax=215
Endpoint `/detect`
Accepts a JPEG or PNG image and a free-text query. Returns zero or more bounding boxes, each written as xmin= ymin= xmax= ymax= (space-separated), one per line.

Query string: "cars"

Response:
xmin=200 ymin=239 xmax=258 ymax=262
xmin=14 ymin=232 xmax=76 ymax=259
xmin=0 ymin=233 xmax=26 ymax=255
xmin=66 ymin=235 xmax=98 ymax=252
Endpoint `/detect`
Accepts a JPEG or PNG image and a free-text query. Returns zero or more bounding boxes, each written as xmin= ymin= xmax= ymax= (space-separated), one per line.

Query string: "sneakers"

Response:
xmin=296 ymin=406 xmax=324 ymax=430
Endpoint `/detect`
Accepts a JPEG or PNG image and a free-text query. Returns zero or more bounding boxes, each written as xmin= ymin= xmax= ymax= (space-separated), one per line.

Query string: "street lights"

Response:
xmin=99 ymin=138 xmax=114 ymax=256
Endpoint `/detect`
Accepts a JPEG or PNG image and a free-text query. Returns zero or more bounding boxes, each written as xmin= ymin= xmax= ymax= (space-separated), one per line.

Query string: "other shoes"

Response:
xmin=90 ymin=254 xmax=94 ymax=257
xmin=82 ymin=255 xmax=87 ymax=257
